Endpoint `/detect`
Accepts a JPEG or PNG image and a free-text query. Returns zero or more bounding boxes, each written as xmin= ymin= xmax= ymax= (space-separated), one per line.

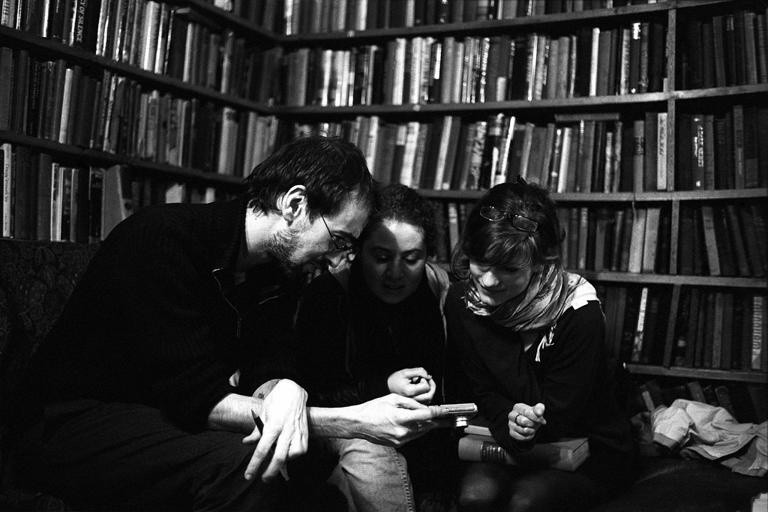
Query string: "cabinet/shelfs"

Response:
xmin=0 ymin=1 xmax=768 ymax=391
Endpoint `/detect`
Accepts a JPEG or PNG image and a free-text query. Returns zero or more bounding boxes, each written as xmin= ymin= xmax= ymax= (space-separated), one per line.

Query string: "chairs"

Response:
xmin=0 ymin=237 xmax=114 ymax=512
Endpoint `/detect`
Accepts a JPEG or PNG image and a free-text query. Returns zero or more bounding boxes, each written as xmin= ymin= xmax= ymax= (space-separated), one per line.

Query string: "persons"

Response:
xmin=14 ymin=125 xmax=639 ymax=512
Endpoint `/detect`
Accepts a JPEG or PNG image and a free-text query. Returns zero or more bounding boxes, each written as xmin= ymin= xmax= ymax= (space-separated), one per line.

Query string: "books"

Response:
xmin=2 ymin=0 xmax=767 ymax=422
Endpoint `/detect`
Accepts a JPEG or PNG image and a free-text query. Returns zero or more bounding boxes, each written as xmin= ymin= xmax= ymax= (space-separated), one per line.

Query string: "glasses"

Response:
xmin=319 ymin=211 xmax=362 ymax=263
xmin=479 ymin=205 xmax=540 ymax=233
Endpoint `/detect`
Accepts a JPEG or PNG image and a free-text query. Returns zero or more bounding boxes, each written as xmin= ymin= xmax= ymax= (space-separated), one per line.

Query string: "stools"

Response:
xmin=421 ymin=445 xmax=768 ymax=512
